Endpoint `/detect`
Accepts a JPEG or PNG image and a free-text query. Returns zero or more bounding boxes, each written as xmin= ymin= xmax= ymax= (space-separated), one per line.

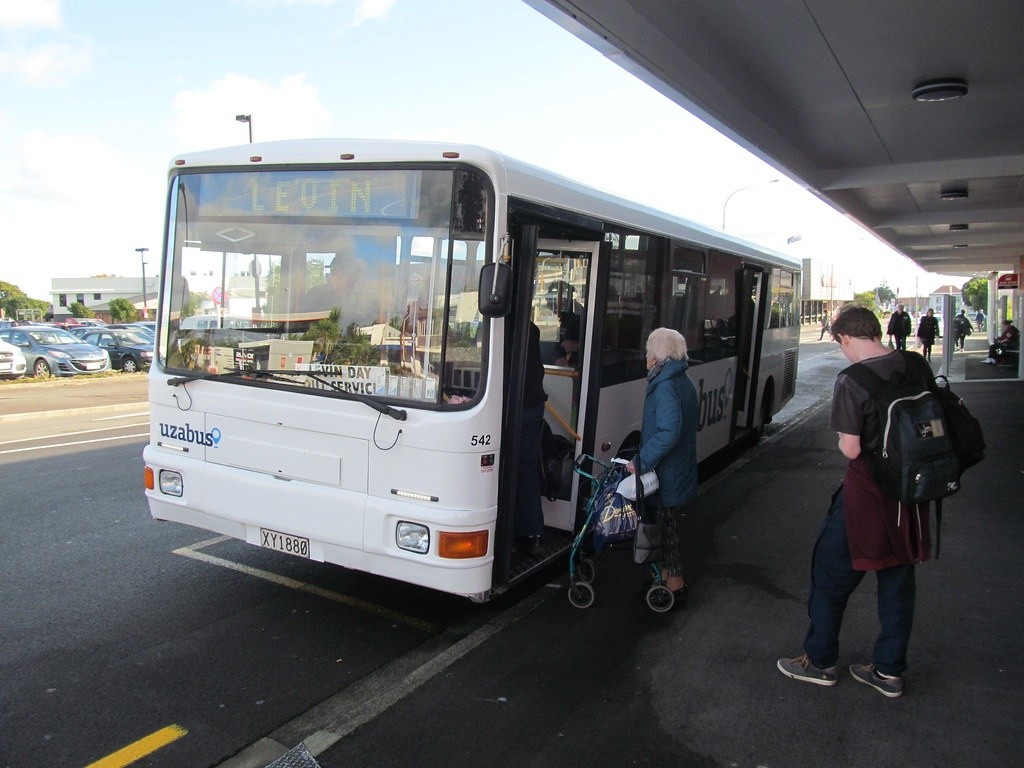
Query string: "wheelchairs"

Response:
xmin=544 ymin=453 xmax=676 ymax=613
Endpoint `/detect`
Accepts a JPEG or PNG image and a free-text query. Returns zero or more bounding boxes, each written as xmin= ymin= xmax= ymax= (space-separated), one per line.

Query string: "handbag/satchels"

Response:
xmin=632 ymin=522 xmax=664 ymax=564
xmin=592 ymin=465 xmax=637 ymax=552
xmin=889 ymin=336 xmax=894 ymax=350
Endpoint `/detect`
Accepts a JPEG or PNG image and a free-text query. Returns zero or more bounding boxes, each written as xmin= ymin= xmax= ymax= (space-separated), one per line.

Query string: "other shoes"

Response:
xmin=981 ymin=358 xmax=996 ymax=365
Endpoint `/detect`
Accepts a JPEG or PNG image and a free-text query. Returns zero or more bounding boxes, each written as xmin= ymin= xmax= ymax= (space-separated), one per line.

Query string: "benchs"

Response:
xmin=995 ymin=337 xmax=1020 ymax=360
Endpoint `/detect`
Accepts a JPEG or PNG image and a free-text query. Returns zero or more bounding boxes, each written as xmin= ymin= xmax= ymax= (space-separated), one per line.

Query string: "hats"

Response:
xmin=324 ymin=255 xmax=360 ymax=268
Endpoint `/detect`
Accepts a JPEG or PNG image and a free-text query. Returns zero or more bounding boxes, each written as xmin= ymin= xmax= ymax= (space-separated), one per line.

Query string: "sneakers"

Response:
xmin=850 ymin=664 xmax=903 ymax=697
xmin=777 ymin=654 xmax=838 ymax=686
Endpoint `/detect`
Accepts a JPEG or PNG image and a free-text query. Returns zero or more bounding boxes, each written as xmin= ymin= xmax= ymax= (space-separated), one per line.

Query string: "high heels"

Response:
xmin=647 ymin=574 xmax=665 ymax=588
xmin=649 ymin=581 xmax=688 ymax=605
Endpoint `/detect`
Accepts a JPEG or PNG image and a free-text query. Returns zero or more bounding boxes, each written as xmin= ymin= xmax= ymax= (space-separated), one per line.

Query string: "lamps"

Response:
xmin=950 ymin=224 xmax=967 ymax=231
xmin=940 ymin=190 xmax=967 ymax=201
xmin=913 ymin=76 xmax=969 ymax=103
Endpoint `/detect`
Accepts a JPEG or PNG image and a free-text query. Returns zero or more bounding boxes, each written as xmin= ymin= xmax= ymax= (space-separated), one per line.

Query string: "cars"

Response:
xmin=79 ymin=329 xmax=155 ymax=374
xmin=907 ymin=312 xmax=986 ymax=339
xmin=1 ymin=317 xmax=156 ymax=344
xmin=0 ymin=325 xmax=111 ymax=381
xmin=0 ymin=338 xmax=27 ymax=380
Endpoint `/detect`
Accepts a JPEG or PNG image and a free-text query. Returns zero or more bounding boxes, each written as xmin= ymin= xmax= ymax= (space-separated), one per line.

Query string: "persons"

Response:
xmin=887 ymin=304 xmax=911 ymax=350
xmin=955 ymin=309 xmax=974 ymax=352
xmin=449 ymin=316 xmax=545 ymax=541
xmin=976 ymin=309 xmax=985 ymax=332
xmin=777 ymin=306 xmax=936 ymax=697
xmin=917 ymin=308 xmax=939 ymax=362
xmin=817 ymin=309 xmax=831 ymax=341
xmin=981 ymin=320 xmax=1018 ymax=364
xmin=537 ymin=284 xmax=642 ymax=383
xmin=627 ymin=327 xmax=699 ymax=602
xmin=293 ymin=255 xmax=379 ymax=333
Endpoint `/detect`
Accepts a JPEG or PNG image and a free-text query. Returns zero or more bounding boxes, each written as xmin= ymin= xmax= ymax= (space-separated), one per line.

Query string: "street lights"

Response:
xmin=135 ymin=247 xmax=150 ymax=320
xmin=236 ymin=114 xmax=252 ymax=143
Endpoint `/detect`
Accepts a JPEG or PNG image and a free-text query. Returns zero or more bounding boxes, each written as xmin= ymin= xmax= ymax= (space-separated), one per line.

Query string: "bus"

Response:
xmin=141 ymin=138 xmax=802 ymax=605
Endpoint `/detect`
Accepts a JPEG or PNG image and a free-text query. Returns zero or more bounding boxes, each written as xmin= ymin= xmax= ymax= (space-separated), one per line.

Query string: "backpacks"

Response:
xmin=838 ymin=350 xmax=986 ymax=506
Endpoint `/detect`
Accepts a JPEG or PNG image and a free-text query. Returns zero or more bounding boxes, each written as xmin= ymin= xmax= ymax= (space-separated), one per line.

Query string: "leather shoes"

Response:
xmin=513 ymin=534 xmax=544 ymax=553
